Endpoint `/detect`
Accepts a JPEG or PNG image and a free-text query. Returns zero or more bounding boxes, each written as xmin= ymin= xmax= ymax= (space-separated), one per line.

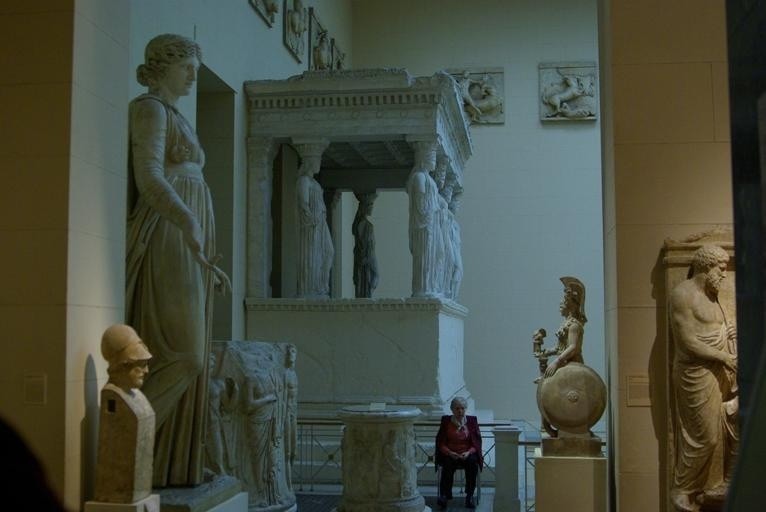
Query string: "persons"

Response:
xmin=668 ymin=244 xmax=738 ymax=512
xmin=203 ymin=341 xmax=299 ymax=510
xmin=125 ymin=34 xmax=235 ymax=489
xmin=352 ymin=191 xmax=381 ymax=298
xmin=435 ymin=395 xmax=484 ymax=509
xmin=97 ymin=324 xmax=157 ymax=504
xmin=406 ymin=141 xmax=464 ymax=301
xmin=293 ymin=156 xmax=335 ymax=299
xmin=530 ymin=275 xmax=588 ymax=438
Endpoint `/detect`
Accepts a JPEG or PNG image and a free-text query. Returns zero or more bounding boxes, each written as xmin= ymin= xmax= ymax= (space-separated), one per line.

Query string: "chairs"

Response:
xmin=438 ymin=417 xmax=483 ymax=505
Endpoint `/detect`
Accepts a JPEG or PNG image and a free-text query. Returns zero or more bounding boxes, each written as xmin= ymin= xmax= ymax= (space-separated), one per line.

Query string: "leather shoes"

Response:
xmin=466 ymin=495 xmax=475 ymax=508
xmin=437 ymin=496 xmax=448 ymax=508
